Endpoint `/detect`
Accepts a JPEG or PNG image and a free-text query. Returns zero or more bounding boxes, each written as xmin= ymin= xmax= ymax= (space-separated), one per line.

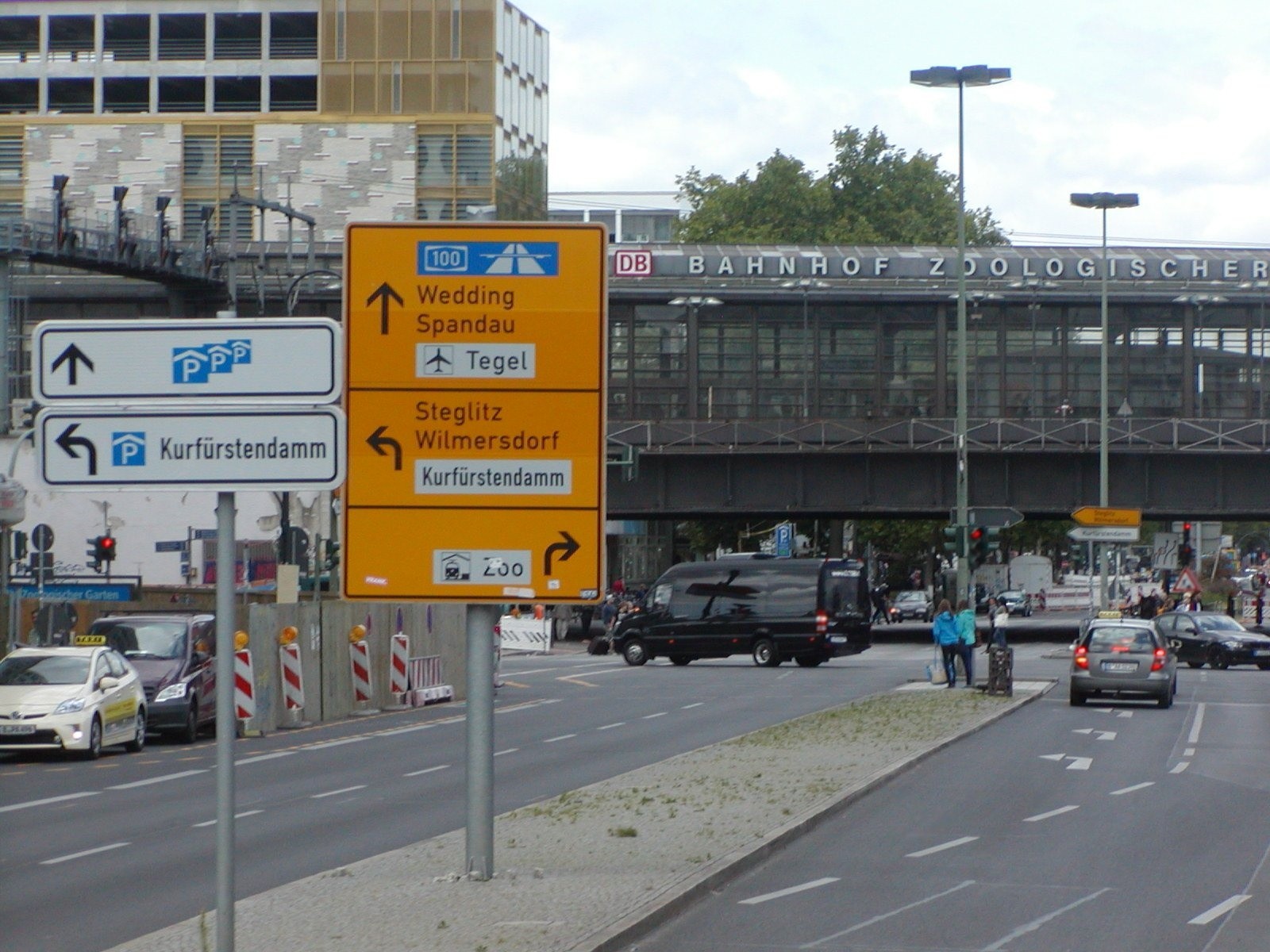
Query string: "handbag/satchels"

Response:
xmin=994 ymin=608 xmax=1009 ymax=628
xmin=926 ymin=663 xmax=948 ymax=684
xmin=971 ymin=629 xmax=982 ymax=647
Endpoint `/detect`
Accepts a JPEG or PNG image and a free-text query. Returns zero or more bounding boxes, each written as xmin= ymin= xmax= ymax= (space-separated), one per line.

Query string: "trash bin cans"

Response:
xmin=989 ymin=646 xmax=1014 ymax=697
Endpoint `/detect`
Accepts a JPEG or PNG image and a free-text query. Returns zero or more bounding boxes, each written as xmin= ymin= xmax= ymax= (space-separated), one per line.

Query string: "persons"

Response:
xmin=956 ymin=599 xmax=977 ymax=688
xmin=1104 ymin=576 xmax=1204 ymax=621
xmin=868 ymin=578 xmax=1033 ymax=655
xmin=506 ymin=573 xmax=649 ymax=657
xmin=933 ymin=599 xmax=960 ymax=689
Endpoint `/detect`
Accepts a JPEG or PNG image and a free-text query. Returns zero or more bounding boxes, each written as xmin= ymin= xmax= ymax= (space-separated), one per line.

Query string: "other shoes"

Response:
xmin=965 ymin=683 xmax=972 ymax=687
xmin=949 ymin=683 xmax=955 ymax=688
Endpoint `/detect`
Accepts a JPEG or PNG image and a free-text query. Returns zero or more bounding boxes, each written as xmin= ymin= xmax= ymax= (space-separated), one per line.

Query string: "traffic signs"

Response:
xmin=29 ymin=317 xmax=346 ymax=492
xmin=343 ymin=220 xmax=606 ymax=604
xmin=1066 ymin=507 xmax=1143 ymax=541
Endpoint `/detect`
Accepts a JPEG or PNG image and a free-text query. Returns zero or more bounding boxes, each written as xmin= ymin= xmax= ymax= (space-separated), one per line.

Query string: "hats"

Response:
xmin=880 ymin=583 xmax=889 ymax=590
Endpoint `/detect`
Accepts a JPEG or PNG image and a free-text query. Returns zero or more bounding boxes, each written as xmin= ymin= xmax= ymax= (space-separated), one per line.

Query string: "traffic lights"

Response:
xmin=84 ymin=536 xmax=116 ymax=570
xmin=1178 ymin=521 xmax=1192 ymax=564
xmin=945 ymin=523 xmax=1003 ymax=568
xmin=17 ymin=530 xmax=29 ymax=558
xmin=323 ymin=538 xmax=341 ymax=570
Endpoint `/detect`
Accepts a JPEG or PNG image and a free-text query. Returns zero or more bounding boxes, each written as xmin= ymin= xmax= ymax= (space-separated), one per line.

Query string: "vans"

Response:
xmin=84 ymin=610 xmax=219 ymax=744
xmin=608 ymin=558 xmax=872 ymax=669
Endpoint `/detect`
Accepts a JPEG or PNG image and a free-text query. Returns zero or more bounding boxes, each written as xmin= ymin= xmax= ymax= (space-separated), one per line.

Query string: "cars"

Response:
xmin=996 ymin=589 xmax=1033 ymax=617
xmin=1069 ymin=618 xmax=1182 ymax=709
xmin=0 ymin=644 xmax=150 ymax=760
xmin=1150 ymin=610 xmax=1270 ymax=671
xmin=890 ymin=590 xmax=937 ymax=624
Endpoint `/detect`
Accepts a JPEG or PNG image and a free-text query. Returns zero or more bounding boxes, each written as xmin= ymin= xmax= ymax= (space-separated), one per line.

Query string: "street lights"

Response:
xmin=1071 ymin=191 xmax=1140 ymax=613
xmin=908 ymin=65 xmax=1013 ymax=609
